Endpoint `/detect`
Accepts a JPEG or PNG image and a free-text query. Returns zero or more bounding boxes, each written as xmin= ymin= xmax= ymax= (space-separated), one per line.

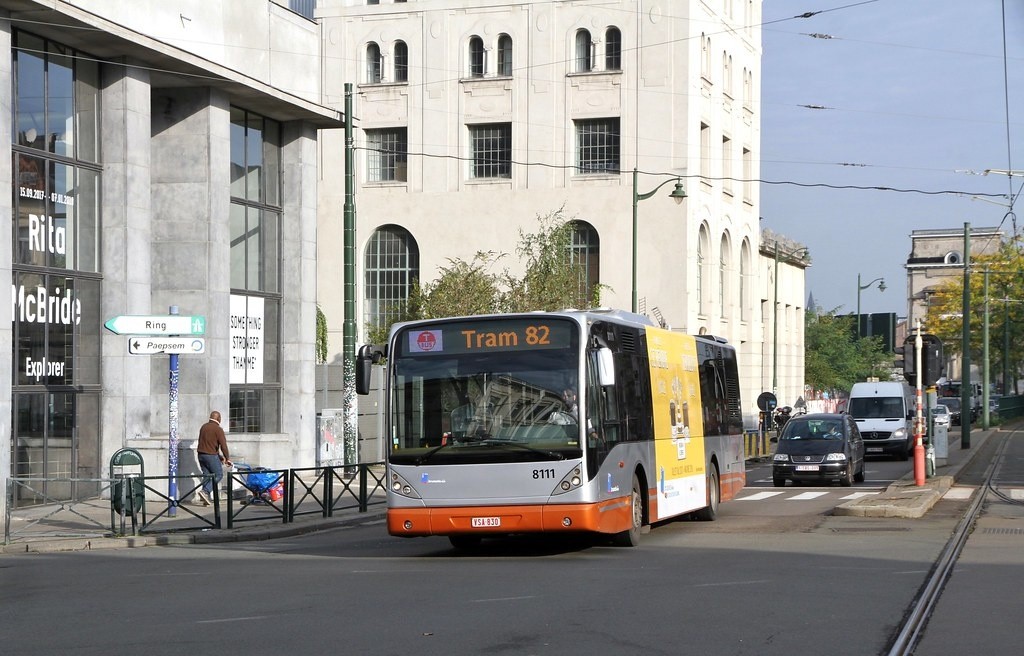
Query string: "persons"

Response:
xmin=823 ymin=423 xmax=843 ymax=440
xmin=548 ymin=387 xmax=599 ymax=439
xmin=197 ymin=411 xmax=232 ymax=507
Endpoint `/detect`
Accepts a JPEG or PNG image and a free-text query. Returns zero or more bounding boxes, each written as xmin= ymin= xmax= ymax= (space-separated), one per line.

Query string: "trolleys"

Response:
xmin=227 ymin=457 xmax=282 ymax=508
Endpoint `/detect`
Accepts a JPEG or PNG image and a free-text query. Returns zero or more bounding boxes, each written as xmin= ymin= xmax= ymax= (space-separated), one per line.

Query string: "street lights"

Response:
xmin=770 ymin=240 xmax=814 ymax=399
xmin=856 ymin=274 xmax=888 ymax=340
xmin=630 ymin=167 xmax=689 ymax=313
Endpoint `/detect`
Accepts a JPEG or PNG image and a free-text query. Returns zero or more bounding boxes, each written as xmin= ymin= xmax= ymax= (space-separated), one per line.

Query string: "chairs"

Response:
xmin=450 ymin=403 xmax=495 ymax=444
xmin=791 ymin=422 xmax=809 ymax=439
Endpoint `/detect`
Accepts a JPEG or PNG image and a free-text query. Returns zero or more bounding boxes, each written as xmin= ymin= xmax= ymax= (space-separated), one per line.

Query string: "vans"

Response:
xmin=845 ymin=379 xmax=918 ymax=461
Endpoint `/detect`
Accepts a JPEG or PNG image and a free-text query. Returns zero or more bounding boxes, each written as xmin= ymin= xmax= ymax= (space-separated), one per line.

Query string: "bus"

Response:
xmin=355 ymin=307 xmax=748 ymax=553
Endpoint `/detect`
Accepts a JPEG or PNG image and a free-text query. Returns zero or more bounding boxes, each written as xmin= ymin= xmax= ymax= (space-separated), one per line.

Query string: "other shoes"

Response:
xmin=199 ymin=490 xmax=211 ymax=505
xmin=202 ymin=502 xmax=210 ymax=508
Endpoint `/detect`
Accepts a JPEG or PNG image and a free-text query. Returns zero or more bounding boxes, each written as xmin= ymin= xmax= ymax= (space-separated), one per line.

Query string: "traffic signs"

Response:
xmin=104 ymin=315 xmax=206 ymax=337
xmin=129 ymin=337 xmax=207 ymax=355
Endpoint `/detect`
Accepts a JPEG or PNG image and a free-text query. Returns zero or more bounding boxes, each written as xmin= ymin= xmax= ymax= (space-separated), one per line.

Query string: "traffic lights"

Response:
xmin=893 ymin=344 xmax=913 ymax=372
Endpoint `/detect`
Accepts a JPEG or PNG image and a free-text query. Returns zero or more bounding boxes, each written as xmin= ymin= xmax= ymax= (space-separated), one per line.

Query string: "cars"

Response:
xmin=769 ymin=411 xmax=867 ymax=487
xmin=938 ymin=380 xmax=999 ymax=426
xmin=924 ymin=404 xmax=953 ymax=432
